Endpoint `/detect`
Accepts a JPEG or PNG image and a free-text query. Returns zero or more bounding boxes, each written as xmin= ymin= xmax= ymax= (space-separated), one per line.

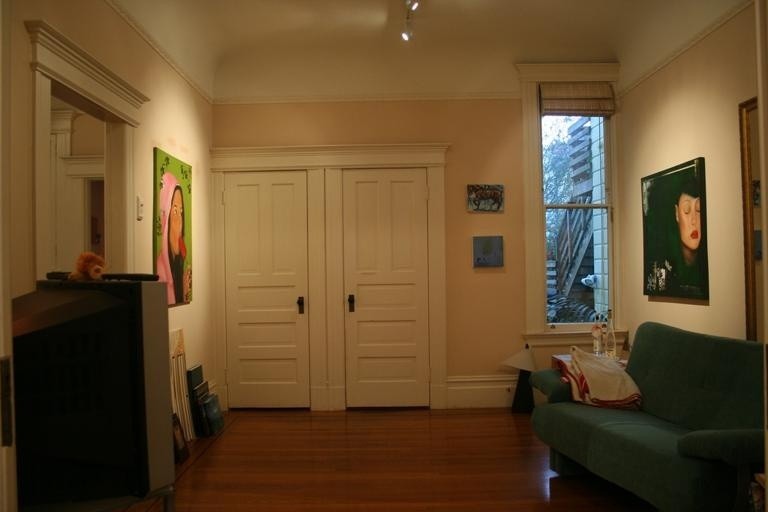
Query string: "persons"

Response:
xmin=663 ymin=175 xmax=703 ymax=287
xmin=158 ymin=172 xmax=192 ymax=306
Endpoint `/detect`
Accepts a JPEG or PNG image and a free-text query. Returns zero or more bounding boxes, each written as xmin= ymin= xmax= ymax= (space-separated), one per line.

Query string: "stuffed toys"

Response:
xmin=68 ymin=252 xmax=105 ymax=281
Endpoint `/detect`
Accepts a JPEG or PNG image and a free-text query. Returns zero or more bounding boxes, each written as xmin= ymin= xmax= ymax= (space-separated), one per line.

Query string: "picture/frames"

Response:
xmin=639 ymin=155 xmax=710 ymax=302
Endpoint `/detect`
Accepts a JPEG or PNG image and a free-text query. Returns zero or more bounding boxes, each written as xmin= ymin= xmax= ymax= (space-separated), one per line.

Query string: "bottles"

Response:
xmin=605 ymin=309 xmax=616 ymax=359
xmin=592 ymin=313 xmax=603 ymax=359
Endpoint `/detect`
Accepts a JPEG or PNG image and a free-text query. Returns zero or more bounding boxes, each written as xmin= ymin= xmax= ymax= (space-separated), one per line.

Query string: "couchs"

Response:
xmin=527 ymin=319 xmax=766 ymax=512
